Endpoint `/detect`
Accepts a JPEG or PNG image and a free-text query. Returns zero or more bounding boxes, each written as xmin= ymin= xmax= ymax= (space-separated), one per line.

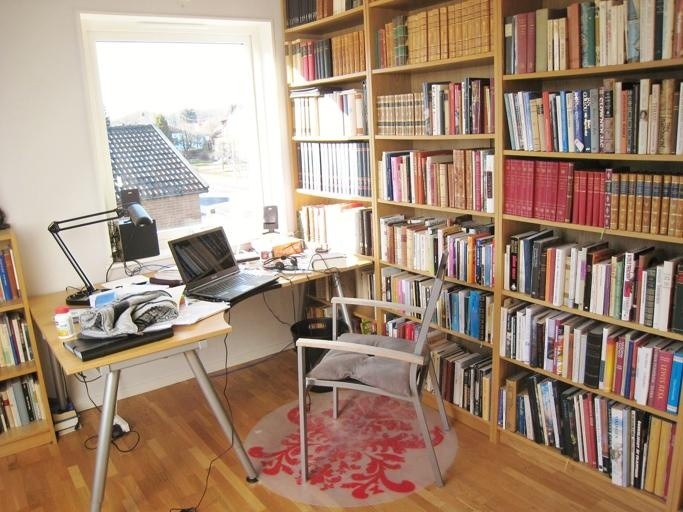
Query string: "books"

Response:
xmin=374 ymin=1 xmax=491 ymax=69
xmin=285 ymin=30 xmax=367 ymax=84
xmin=502 ymin=79 xmax=681 ymax=151
xmin=1 ymin=244 xmax=41 ymax=439
xmin=501 ymin=294 xmax=679 ymax=414
xmin=502 ymin=156 xmax=682 ymax=237
xmin=297 ymin=201 xmax=373 ymax=256
xmin=289 ymin=87 xmax=367 ymax=139
xmin=401 ymin=319 xmax=490 ymax=421
xmin=63 ymin=328 xmax=175 ymax=362
xmin=295 ymin=141 xmax=373 ymax=197
xmin=504 ymin=230 xmax=681 ymax=333
xmin=503 ymin=1 xmax=680 ymax=75
xmin=306 ymin=306 xmax=376 ymax=342
xmin=499 ymin=367 xmax=676 ymax=499
xmin=375 ymin=77 xmax=496 ymax=136
xmin=148 ymin=267 xmax=185 ymax=286
xmin=379 ymin=214 xmax=496 ymax=289
xmin=231 ymin=240 xmax=261 ymax=262
xmin=377 ymin=147 xmax=495 ymax=214
xmin=50 ymin=398 xmax=83 ymax=437
xmin=302 ymin=265 xmax=375 ymax=318
xmin=381 ymin=265 xmax=493 ymax=345
xmin=286 ymin=3 xmax=362 ymax=29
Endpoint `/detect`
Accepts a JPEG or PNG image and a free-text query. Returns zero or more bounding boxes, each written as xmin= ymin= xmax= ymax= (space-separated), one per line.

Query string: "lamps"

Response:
xmin=46 ymin=201 xmax=152 ymax=305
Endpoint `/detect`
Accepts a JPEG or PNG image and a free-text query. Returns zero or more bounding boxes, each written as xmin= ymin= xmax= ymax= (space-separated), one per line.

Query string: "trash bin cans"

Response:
xmin=291 ymin=317 xmax=348 ymax=393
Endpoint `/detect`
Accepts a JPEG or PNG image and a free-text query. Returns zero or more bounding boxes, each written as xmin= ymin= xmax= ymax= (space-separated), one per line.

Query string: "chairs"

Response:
xmin=294 ymin=252 xmax=450 ymax=488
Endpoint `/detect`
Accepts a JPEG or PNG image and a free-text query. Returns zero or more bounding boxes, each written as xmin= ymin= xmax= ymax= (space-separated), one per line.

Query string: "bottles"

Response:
xmin=54 ymin=306 xmax=75 ymax=340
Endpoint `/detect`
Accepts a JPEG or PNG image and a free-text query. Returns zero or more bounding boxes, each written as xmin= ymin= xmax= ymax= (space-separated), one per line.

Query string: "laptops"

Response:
xmin=167 ymin=226 xmax=280 ymax=302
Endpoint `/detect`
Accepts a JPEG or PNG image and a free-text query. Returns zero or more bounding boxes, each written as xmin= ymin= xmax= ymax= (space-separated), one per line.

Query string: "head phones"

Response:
xmin=263 ymin=255 xmax=297 ymax=270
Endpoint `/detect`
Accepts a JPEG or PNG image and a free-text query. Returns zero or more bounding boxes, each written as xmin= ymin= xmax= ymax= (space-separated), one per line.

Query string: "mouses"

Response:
xmin=284 ymin=265 xmax=298 ymax=270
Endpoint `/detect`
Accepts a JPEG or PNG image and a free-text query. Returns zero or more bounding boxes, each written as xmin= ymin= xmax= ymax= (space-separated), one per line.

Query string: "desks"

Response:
xmin=26 ymin=234 xmax=373 ymax=511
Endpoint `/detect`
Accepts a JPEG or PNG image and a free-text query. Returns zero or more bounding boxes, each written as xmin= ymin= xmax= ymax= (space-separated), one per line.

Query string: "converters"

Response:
xmin=111 ymin=424 xmax=123 ymax=438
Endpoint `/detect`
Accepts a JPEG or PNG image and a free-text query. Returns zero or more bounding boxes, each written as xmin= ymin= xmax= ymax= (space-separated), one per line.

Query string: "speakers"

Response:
xmin=262 ymin=206 xmax=281 ymax=235
xmin=121 ymin=188 xmax=142 ymax=223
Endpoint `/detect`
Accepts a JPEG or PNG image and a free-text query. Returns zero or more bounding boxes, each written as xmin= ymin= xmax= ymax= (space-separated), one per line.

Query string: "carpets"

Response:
xmin=242 ymin=387 xmax=458 ymax=508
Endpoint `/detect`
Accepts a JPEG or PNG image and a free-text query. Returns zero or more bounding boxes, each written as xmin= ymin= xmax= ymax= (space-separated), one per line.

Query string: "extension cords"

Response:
xmin=113 ymin=415 xmax=130 ymax=433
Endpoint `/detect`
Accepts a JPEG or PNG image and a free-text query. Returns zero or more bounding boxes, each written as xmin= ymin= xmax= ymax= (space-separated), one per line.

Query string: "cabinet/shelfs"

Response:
xmin=279 ymin=0 xmax=683 ymax=511
xmin=0 ymin=227 xmax=57 ymax=459
